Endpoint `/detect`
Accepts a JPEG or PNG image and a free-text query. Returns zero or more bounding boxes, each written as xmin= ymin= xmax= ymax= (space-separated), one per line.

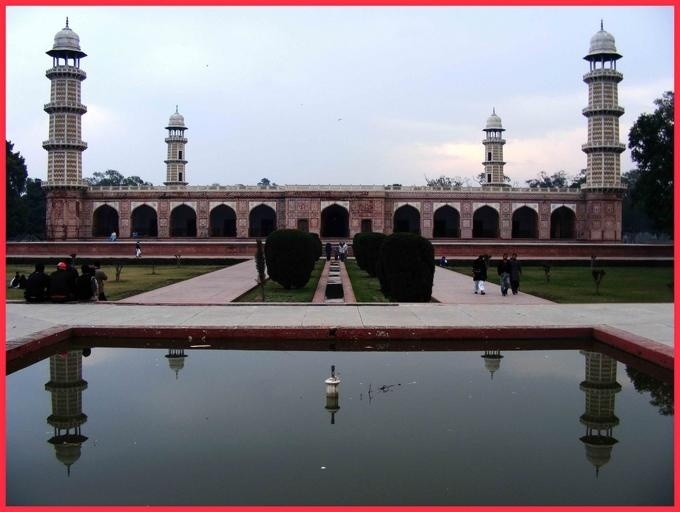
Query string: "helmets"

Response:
xmin=57 ymin=261 xmax=66 ymax=269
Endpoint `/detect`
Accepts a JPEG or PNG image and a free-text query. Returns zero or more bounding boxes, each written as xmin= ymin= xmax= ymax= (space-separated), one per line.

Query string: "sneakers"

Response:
xmin=475 ymin=291 xmax=486 ymax=295
xmin=502 ymin=289 xmax=518 ymax=296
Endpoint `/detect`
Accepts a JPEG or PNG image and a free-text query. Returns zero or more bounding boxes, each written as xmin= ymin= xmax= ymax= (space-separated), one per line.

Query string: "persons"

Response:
xmin=472 ymin=255 xmax=487 ymax=295
xmin=7 ymin=262 xmax=108 ymax=304
xmin=496 ymin=252 xmax=514 ymax=296
xmin=325 ymin=241 xmax=349 ymax=262
xmin=509 ymin=252 xmax=522 ymax=295
xmin=135 ymin=241 xmax=142 ymax=257
xmin=440 ymin=256 xmax=448 ymax=267
